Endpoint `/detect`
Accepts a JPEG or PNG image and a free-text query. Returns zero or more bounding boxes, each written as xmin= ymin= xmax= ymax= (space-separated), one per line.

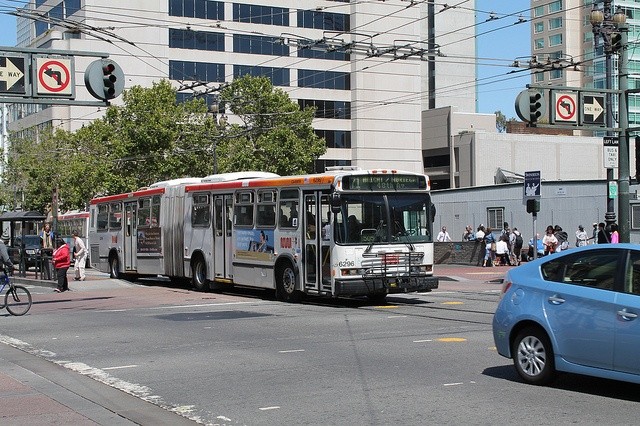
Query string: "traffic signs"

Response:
xmin=0 ymin=51 xmax=29 ymax=97
xmin=579 ymin=93 xmax=606 ymax=126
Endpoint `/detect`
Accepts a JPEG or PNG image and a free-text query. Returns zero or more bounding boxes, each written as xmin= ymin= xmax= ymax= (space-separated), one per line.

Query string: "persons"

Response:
xmin=576 ymin=225 xmax=589 ymax=247
xmin=348 ymin=215 xmax=362 ymax=236
xmin=480 ymin=226 xmax=485 ymax=233
xmin=506 ymin=229 xmax=510 ymax=236
xmin=464 ymin=227 xmax=476 ymax=241
xmin=553 ymin=225 xmax=568 ymax=250
xmin=542 ymin=225 xmax=558 ymax=256
xmin=509 ymin=227 xmax=524 ymax=266
xmin=436 ymin=226 xmax=453 ymax=242
xmin=526 ymin=233 xmax=543 ymax=262
xmin=258 ymin=230 xmax=267 ymax=253
xmin=0 ymin=227 xmax=18 ymax=279
xmin=589 ymin=222 xmax=600 ymax=244
xmin=38 ymin=222 xmax=53 ymax=248
xmin=478 ymin=227 xmax=497 ymax=267
xmin=479 ymin=224 xmax=483 ymax=232
xmin=462 ymin=225 xmax=471 ymax=242
xmin=475 ymin=226 xmax=486 ymax=241
xmin=52 ymin=238 xmax=71 ymax=293
xmin=598 ymin=223 xmax=611 ymax=244
xmin=192 ymin=203 xmax=315 ymax=227
xmin=500 ymin=222 xmax=510 ymax=235
xmin=72 ymin=230 xmax=88 ymax=281
xmin=322 ymin=225 xmax=330 ymax=240
xmin=610 ymin=224 xmax=619 ymax=244
xmin=495 ymin=236 xmax=513 ymax=266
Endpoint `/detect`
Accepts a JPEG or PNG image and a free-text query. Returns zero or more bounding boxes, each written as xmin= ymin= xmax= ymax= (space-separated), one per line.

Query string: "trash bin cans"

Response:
xmin=41 ymin=256 xmax=54 ymax=280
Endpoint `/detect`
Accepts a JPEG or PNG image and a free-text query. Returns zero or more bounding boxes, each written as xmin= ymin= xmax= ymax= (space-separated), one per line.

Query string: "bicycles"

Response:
xmin=0 ymin=266 xmax=32 ymax=316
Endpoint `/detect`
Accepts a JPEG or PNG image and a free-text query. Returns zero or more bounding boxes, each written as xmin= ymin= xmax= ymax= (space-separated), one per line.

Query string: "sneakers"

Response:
xmin=73 ymin=277 xmax=85 ymax=281
xmin=54 ymin=287 xmax=64 ymax=293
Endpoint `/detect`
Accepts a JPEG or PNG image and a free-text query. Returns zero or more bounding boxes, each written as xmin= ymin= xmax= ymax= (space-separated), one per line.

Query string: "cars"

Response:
xmin=7 ymin=235 xmax=43 ymax=271
xmin=493 ymin=243 xmax=640 ymax=384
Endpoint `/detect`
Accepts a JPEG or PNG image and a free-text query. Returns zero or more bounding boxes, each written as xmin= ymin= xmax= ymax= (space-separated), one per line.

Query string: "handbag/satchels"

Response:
xmin=528 ymin=245 xmax=533 ymax=257
xmin=576 ymin=240 xmax=580 ymax=246
xmin=491 ymin=239 xmax=496 ymax=252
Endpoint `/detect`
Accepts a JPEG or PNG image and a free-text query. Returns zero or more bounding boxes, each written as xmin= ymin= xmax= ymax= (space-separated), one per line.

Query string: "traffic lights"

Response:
xmin=527 ymin=199 xmax=540 ymax=213
xmin=85 ymin=59 xmax=125 ymax=100
xmin=530 ymin=93 xmax=541 ymax=122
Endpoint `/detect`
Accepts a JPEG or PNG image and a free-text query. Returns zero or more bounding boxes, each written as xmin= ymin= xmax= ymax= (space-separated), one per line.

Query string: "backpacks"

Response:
xmin=513 ymin=232 xmax=523 ymax=248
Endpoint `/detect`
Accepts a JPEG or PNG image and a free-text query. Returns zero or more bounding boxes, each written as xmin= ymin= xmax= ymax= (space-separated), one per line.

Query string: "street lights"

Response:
xmin=210 ymin=100 xmax=219 ymax=174
xmin=590 ymin=3 xmax=626 ymax=240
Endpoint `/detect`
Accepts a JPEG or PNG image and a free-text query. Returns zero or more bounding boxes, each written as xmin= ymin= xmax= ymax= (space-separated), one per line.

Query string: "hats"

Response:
xmin=56 ymin=239 xmax=65 ymax=244
xmin=593 ymin=223 xmax=597 ymax=227
xmin=466 ymin=225 xmax=471 ymax=230
xmin=546 ymin=226 xmax=555 ymax=231
xmin=1 ymin=232 xmax=10 ymax=240
xmin=537 ymin=233 xmax=541 ymax=239
xmin=479 ymin=224 xmax=485 ymax=229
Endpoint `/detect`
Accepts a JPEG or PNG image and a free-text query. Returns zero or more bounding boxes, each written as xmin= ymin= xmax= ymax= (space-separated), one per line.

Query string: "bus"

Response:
xmin=88 ymin=170 xmax=438 ymax=301
xmin=50 ymin=210 xmax=89 ymax=267
